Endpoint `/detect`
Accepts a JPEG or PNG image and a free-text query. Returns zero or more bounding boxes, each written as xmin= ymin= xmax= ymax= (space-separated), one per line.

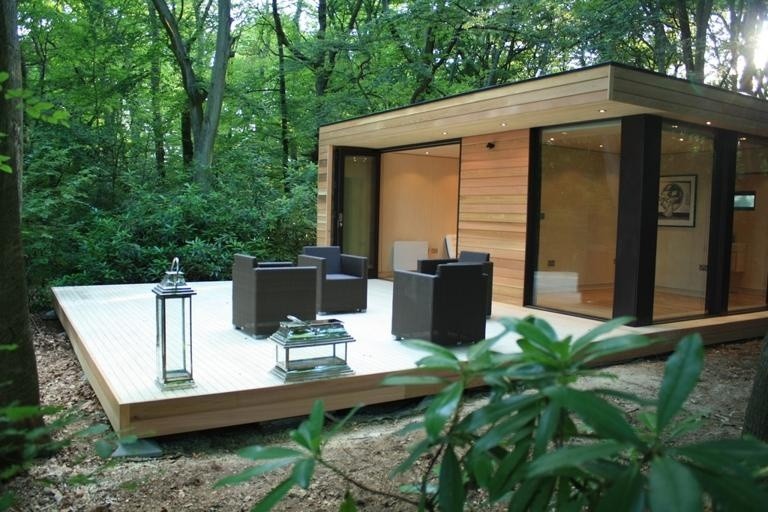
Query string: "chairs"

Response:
xmin=417 ymin=251 xmax=493 ymax=318
xmin=298 ymin=246 xmax=368 ymax=316
xmin=232 ymin=253 xmax=317 ymax=340
xmin=392 ymin=264 xmax=489 ymax=345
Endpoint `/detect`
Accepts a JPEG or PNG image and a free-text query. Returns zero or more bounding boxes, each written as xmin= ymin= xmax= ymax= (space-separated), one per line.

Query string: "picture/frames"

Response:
xmin=656 ymin=174 xmax=697 ymax=227
